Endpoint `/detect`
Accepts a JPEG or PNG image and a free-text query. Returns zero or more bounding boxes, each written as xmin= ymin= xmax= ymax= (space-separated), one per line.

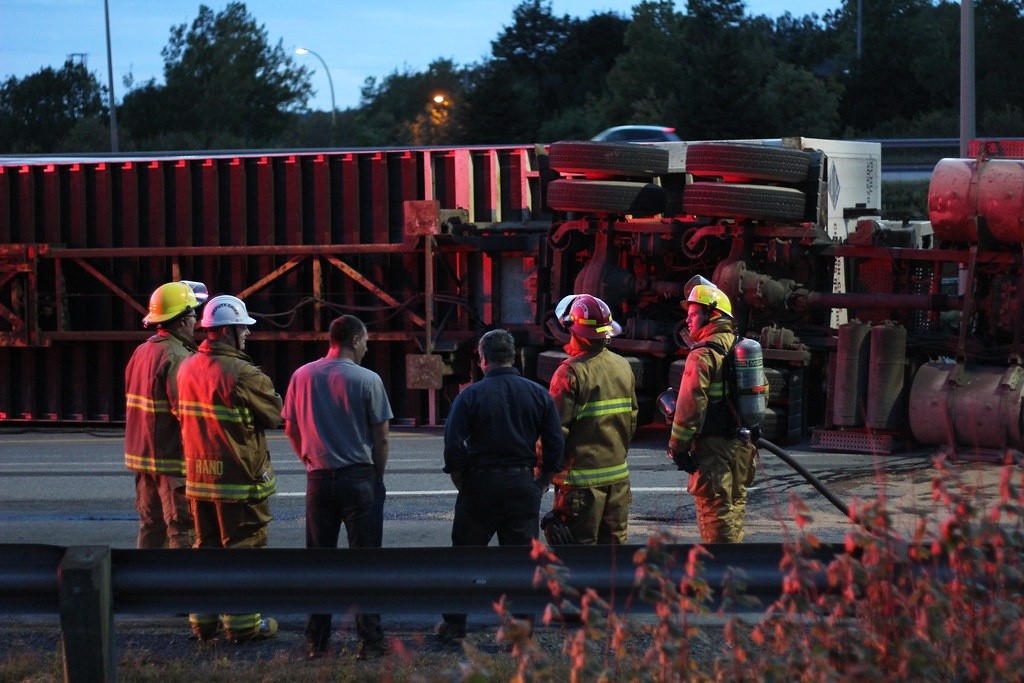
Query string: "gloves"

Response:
xmin=540 ymin=511 xmax=574 ymax=547
xmin=672 ymin=449 xmax=700 ymax=475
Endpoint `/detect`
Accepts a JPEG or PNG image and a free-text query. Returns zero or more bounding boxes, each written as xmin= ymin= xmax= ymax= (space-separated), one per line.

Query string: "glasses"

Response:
xmin=477 ymin=359 xmax=482 ymax=367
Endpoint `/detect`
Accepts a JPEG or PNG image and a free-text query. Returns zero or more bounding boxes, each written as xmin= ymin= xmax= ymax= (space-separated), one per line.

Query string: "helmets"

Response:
xmin=680 ymin=284 xmax=734 ymax=319
xmin=142 ymin=282 xmax=202 ymax=324
xmin=201 ymin=295 xmax=257 ymax=327
xmin=563 ymin=294 xmax=622 ymax=340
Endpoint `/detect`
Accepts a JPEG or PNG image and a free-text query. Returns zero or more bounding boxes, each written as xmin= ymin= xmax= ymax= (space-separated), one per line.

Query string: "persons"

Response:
xmin=444 ymin=331 xmax=564 ymax=547
xmin=535 ymin=294 xmax=639 ymax=544
xmin=177 ymin=295 xmax=283 ymax=547
xmin=125 ymin=281 xmax=209 ymax=548
xmin=281 ymin=315 xmax=393 ymax=546
xmin=668 ymin=274 xmax=756 ymax=543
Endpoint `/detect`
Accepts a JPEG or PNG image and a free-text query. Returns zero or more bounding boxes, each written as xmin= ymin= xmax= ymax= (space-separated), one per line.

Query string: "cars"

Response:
xmin=586 ymin=123 xmax=683 ymax=143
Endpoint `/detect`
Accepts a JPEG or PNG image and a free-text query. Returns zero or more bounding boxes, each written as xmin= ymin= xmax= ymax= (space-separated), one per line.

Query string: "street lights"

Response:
xmin=295 ymin=47 xmax=336 ymax=147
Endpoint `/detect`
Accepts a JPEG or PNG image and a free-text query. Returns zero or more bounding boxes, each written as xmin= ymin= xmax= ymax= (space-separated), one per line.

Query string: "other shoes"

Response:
xmin=308 ymin=639 xmax=328 ymax=657
xmin=357 ymin=638 xmax=396 ymax=659
xmin=434 ymin=621 xmax=466 ymax=640
xmin=256 ymin=618 xmax=278 ymax=638
